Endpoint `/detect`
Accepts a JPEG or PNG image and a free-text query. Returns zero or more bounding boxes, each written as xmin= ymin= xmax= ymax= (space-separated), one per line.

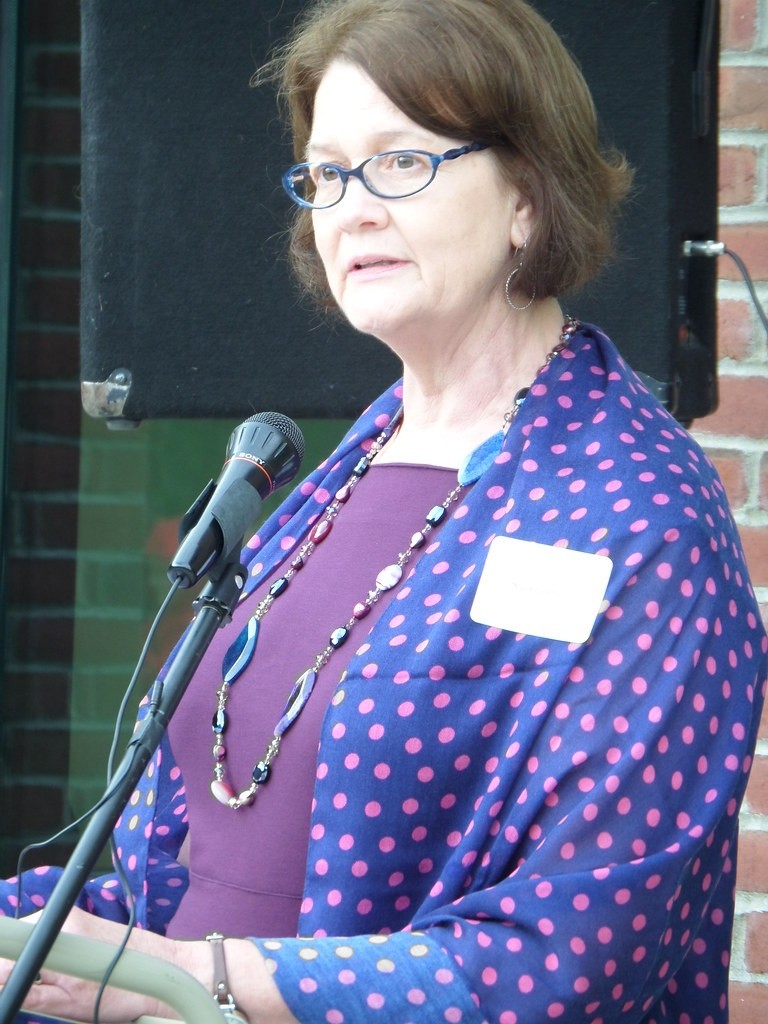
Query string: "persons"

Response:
xmin=1 ymin=1 xmax=768 ymax=1024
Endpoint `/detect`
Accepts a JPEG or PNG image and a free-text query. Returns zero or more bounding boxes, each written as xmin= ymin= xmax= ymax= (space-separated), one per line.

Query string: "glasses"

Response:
xmin=282 ymin=136 xmax=504 ymax=209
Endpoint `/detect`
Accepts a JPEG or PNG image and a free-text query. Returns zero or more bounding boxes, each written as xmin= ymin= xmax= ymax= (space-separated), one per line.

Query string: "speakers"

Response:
xmin=80 ymin=1 xmax=721 ymax=427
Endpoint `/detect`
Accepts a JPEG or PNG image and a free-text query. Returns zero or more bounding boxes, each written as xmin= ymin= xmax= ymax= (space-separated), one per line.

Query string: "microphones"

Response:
xmin=167 ymin=412 xmax=305 ymax=588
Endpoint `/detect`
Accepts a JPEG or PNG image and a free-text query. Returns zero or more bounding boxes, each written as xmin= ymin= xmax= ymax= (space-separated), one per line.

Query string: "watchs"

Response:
xmin=206 ymin=933 xmax=253 ymax=1024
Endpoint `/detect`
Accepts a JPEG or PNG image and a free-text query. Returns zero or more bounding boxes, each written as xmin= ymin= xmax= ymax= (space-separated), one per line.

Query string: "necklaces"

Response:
xmin=210 ymin=314 xmax=578 ymax=810
